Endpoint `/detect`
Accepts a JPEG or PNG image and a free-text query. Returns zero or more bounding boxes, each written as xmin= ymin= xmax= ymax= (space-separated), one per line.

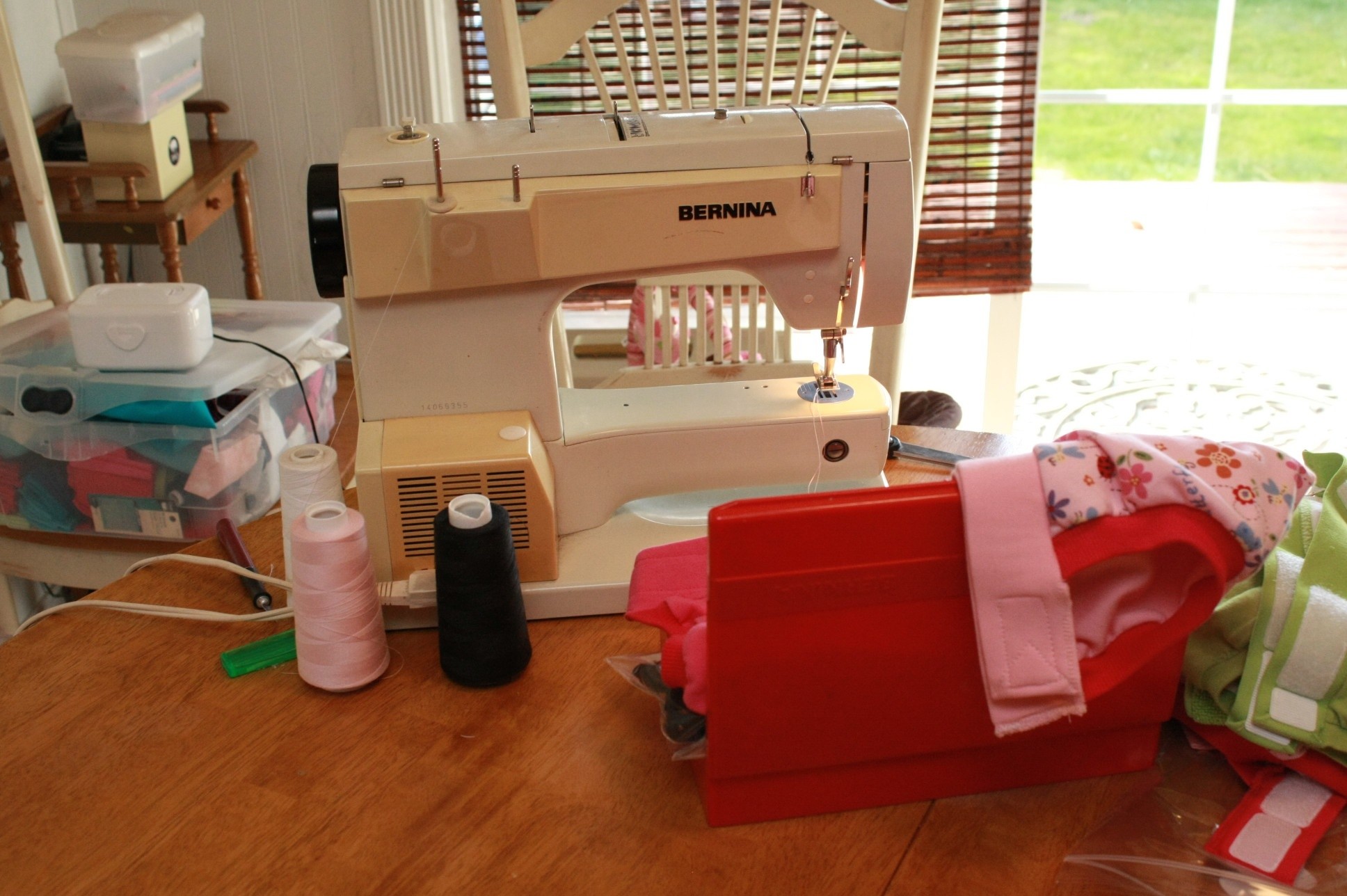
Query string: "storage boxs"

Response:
xmin=50 ymin=6 xmax=209 ymax=124
xmin=79 ymin=102 xmax=196 ymax=204
xmin=0 ymin=286 xmax=347 ymax=540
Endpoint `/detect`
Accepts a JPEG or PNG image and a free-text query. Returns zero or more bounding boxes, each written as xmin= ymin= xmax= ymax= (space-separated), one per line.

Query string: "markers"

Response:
xmin=215 ymin=517 xmax=272 ymax=609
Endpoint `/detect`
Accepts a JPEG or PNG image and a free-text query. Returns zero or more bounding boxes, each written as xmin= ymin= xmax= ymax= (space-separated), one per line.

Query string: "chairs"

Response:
xmin=478 ymin=0 xmax=951 ymax=431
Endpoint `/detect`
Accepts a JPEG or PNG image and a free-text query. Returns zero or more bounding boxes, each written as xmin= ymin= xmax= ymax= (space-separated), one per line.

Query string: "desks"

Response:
xmin=0 ymin=422 xmax=1347 ymax=896
xmin=0 ymin=97 xmax=265 ymax=300
